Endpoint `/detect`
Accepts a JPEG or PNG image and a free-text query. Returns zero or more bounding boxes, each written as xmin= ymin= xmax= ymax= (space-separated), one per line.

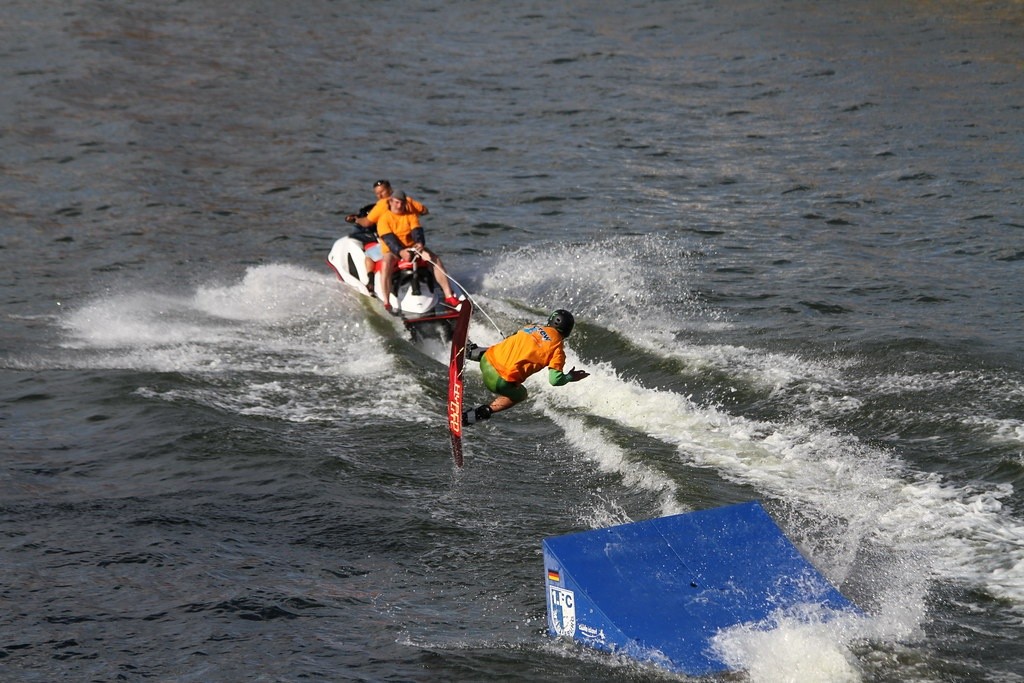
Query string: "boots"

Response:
xmin=466 ymin=339 xmax=485 ymax=361
xmin=463 ymin=405 xmax=493 ymax=427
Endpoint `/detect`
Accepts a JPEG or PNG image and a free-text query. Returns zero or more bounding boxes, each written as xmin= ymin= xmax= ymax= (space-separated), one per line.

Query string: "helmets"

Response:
xmin=548 ymin=309 xmax=574 ymax=336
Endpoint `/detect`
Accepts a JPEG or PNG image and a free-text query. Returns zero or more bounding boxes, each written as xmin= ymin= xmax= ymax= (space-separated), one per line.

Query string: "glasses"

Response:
xmin=373 ymin=181 xmax=384 ymax=186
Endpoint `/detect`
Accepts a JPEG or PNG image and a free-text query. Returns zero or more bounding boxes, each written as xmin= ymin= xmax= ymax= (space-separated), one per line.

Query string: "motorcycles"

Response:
xmin=326 ymin=201 xmax=466 ymax=331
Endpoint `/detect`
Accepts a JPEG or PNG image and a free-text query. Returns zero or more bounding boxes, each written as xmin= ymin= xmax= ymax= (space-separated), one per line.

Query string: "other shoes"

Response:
xmin=445 ymin=296 xmax=462 ymax=306
xmin=385 ymin=303 xmax=392 ymax=313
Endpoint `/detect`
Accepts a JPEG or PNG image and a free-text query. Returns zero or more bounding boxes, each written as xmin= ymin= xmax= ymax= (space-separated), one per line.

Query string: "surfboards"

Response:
xmin=446 ymin=297 xmax=473 ymax=470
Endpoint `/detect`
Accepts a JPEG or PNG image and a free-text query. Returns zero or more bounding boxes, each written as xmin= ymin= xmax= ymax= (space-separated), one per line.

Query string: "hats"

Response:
xmin=392 ymin=190 xmax=406 ymax=201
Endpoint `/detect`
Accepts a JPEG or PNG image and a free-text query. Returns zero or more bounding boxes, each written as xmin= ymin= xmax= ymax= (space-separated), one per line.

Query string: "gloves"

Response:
xmin=565 ymin=366 xmax=591 ymax=382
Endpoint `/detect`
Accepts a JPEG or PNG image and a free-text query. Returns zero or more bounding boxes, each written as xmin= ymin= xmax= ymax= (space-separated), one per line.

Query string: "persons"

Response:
xmin=376 ymin=188 xmax=461 ymax=312
xmin=352 ymin=180 xmax=430 ymax=297
xmin=462 ymin=310 xmax=591 ymax=426
